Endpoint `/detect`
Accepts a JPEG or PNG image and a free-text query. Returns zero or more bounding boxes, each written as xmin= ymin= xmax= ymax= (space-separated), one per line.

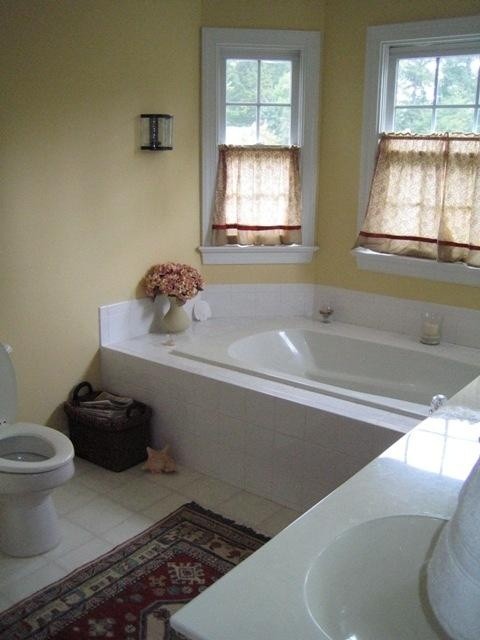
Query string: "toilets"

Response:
xmin=2 ymin=345 xmax=78 ymax=558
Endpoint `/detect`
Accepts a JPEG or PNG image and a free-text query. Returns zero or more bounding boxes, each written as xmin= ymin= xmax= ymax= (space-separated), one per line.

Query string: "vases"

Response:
xmin=162 ymin=295 xmax=190 ymax=332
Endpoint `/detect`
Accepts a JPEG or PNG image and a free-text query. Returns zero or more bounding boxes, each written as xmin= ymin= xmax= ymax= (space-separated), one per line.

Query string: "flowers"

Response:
xmin=141 ymin=261 xmax=207 ymax=306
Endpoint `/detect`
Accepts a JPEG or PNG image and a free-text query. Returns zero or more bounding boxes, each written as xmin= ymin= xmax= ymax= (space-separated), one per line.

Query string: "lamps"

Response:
xmin=140 ymin=113 xmax=173 ymax=151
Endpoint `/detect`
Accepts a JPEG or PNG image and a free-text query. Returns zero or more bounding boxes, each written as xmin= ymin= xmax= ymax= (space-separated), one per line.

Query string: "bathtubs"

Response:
xmin=172 ymin=318 xmax=479 ymax=419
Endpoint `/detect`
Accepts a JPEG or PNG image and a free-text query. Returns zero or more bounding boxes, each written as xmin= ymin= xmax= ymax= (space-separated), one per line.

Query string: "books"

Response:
xmin=77 ymin=391 xmax=134 ymax=431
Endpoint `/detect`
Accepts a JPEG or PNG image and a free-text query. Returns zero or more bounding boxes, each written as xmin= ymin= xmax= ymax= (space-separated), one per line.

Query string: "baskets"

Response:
xmin=61 ymin=380 xmax=156 ymax=474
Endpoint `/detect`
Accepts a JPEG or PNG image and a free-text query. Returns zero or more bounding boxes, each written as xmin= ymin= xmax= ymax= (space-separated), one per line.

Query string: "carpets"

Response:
xmin=0 ymin=500 xmax=271 ymax=640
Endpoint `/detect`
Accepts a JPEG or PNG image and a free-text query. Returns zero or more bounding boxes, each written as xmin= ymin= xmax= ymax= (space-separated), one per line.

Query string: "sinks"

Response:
xmin=305 ymin=513 xmax=456 ymax=640
